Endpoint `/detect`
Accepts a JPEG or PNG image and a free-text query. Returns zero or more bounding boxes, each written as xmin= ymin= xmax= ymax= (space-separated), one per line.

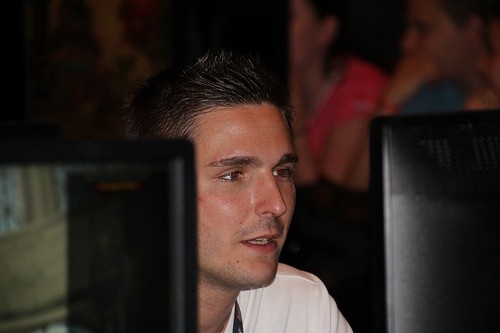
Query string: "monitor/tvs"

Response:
xmin=370 ymin=106 xmax=500 ymax=333
xmin=0 ymin=136 xmax=199 ymax=333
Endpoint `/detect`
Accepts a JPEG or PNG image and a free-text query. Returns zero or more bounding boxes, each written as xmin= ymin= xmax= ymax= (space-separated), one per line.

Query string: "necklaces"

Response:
xmin=232 ymin=300 xmax=244 ymax=333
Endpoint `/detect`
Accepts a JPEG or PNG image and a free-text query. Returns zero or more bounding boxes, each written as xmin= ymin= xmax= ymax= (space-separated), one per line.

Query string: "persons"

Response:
xmin=285 ymin=0 xmax=500 ymax=197
xmin=46 ymin=53 xmax=354 ymax=333
